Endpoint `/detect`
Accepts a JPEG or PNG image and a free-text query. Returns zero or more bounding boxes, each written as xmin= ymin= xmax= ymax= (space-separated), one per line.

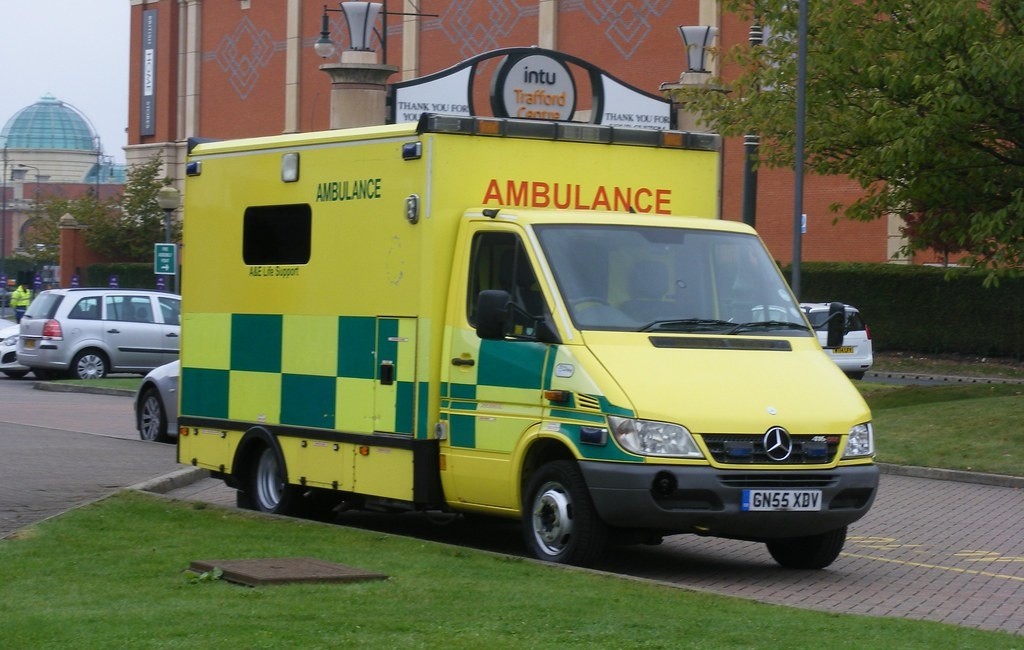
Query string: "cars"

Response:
xmin=726 ymin=303 xmax=873 ymax=380
xmin=0 ymin=297 xmax=174 ymax=379
xmin=133 ymin=359 xmax=181 ymax=442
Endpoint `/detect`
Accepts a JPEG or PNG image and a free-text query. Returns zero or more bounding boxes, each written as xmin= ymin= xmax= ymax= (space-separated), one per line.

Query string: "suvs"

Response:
xmin=16 ymin=288 xmax=182 ymax=381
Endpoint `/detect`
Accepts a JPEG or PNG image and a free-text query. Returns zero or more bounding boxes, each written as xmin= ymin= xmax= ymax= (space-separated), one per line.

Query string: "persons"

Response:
xmin=10 ymin=281 xmax=32 ymax=324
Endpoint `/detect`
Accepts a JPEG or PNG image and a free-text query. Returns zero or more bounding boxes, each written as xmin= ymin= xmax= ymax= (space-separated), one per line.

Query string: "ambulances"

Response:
xmin=177 ymin=46 xmax=880 ymax=569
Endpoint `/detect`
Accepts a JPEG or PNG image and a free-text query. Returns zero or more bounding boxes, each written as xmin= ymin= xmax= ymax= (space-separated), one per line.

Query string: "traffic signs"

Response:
xmin=154 ymin=243 xmax=177 ymax=276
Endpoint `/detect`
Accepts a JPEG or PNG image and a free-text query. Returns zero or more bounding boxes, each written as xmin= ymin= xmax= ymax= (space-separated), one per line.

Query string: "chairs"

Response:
xmin=499 ymin=242 xmax=608 ymax=315
xmin=71 ymin=304 xmax=99 ymax=319
xmin=124 ymin=305 xmax=150 ymax=323
xmin=618 ymin=258 xmax=694 ymax=325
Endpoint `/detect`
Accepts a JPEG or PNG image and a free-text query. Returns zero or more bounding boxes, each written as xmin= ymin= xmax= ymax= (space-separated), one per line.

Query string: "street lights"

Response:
xmin=658 ymin=0 xmax=763 ymax=228
xmin=157 ymin=187 xmax=180 ymax=294
xmin=18 ymin=164 xmax=39 ymax=252
